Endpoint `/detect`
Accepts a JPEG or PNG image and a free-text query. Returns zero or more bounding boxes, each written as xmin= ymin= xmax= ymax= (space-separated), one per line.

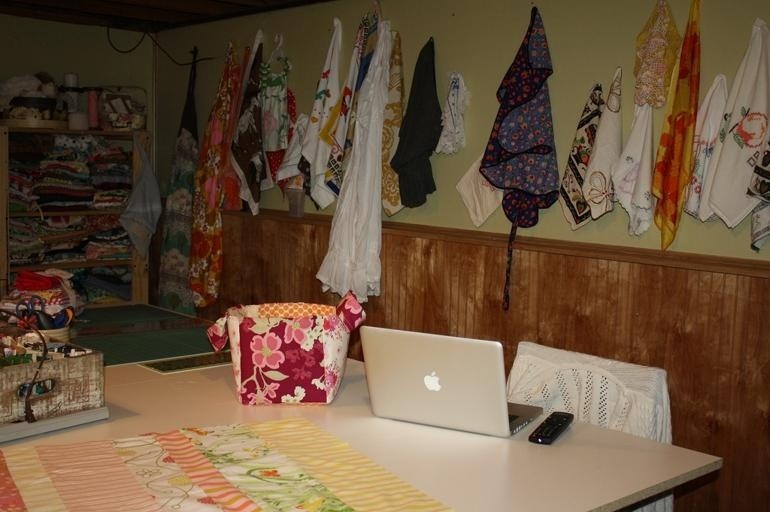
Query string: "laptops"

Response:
xmin=359 ymin=325 xmax=543 ymax=438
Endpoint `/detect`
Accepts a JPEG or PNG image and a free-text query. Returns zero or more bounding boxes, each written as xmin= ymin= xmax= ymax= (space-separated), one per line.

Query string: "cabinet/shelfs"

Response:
xmin=1 ymin=125 xmax=152 ymax=310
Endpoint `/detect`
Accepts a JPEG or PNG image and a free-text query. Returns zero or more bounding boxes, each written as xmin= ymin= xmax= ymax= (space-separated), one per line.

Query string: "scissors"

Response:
xmin=16 ymin=295 xmax=45 ymax=321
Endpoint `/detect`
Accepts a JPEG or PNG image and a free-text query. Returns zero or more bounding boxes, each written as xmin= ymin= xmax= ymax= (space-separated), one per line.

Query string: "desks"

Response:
xmin=0 ymin=304 xmax=723 ymax=512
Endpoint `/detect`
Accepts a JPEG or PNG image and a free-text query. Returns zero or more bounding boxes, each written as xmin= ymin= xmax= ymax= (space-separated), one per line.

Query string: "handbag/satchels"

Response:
xmin=204 ymin=288 xmax=369 ymax=407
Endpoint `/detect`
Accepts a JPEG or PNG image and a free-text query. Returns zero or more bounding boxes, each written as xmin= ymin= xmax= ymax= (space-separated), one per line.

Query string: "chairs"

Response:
xmin=507 ymin=339 xmax=676 ymax=511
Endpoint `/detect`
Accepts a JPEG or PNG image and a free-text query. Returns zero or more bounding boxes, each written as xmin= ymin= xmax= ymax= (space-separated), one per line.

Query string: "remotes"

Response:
xmin=529 ymin=412 xmax=574 ymax=444
